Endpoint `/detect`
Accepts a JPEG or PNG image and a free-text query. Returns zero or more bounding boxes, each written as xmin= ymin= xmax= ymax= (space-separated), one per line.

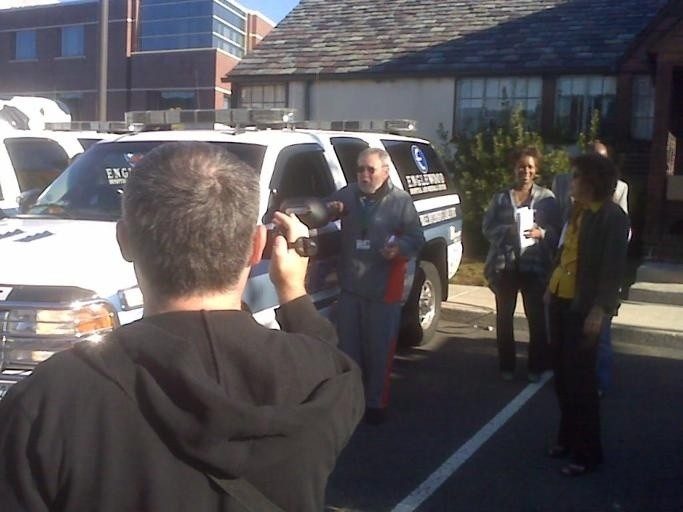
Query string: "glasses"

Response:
xmin=357 ymin=166 xmax=377 ymax=174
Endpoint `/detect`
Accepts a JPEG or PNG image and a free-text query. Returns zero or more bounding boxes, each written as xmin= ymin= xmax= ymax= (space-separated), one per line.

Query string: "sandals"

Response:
xmin=561 ymin=464 xmax=585 ymax=476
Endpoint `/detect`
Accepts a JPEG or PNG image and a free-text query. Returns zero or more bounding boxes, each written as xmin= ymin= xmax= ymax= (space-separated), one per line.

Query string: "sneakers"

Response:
xmin=366 ymin=409 xmax=386 ymax=426
xmin=527 ymin=373 xmax=538 ymax=383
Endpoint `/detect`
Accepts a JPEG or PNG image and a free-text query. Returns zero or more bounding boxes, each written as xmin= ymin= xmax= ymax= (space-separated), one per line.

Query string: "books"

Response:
xmin=514 ymin=204 xmax=536 ymax=250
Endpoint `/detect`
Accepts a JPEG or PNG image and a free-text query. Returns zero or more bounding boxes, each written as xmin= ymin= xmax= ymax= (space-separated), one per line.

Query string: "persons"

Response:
xmin=325 ymin=147 xmax=426 ymax=423
xmin=1 ymin=138 xmax=365 ymax=512
xmin=477 ymin=142 xmax=566 ymax=385
xmin=552 ymin=139 xmax=628 ymax=399
xmin=538 ymin=147 xmax=630 ymax=479
